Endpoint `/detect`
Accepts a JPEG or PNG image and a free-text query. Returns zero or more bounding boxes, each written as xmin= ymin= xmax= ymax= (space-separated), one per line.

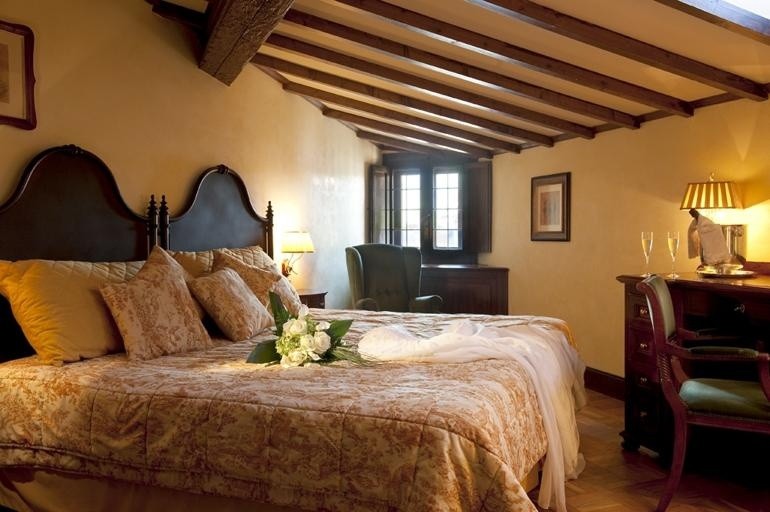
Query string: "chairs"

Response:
xmin=636 ymin=275 xmax=770 ymax=512
xmin=345 ymin=243 xmax=444 ymax=313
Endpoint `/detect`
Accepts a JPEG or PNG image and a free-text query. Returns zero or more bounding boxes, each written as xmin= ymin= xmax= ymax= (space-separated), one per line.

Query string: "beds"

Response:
xmin=0 ymin=143 xmax=588 ymax=512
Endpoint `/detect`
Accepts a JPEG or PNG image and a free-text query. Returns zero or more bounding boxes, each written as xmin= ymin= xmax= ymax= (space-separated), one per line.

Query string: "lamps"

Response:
xmin=679 ymin=172 xmax=745 ymax=211
xmin=279 ymin=228 xmax=314 ymax=292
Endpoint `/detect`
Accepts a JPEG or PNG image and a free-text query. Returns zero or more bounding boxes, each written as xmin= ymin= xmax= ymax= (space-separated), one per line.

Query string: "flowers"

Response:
xmin=244 ymin=289 xmax=372 ymax=372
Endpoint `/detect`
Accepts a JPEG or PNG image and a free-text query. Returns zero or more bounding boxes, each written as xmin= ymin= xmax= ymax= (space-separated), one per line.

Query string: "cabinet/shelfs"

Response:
xmin=420 ymin=266 xmax=509 ymax=314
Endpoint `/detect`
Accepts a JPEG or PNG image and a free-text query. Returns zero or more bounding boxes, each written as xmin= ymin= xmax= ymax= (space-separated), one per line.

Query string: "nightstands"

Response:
xmin=300 ymin=291 xmax=328 ymax=308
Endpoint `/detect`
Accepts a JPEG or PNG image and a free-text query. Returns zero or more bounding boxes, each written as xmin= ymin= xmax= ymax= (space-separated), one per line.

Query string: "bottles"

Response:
xmin=689 ymin=208 xmax=713 ymax=224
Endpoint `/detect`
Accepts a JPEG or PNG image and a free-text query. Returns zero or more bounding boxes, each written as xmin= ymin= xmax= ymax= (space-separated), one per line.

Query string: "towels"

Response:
xmin=685 ymin=215 xmax=729 ymax=265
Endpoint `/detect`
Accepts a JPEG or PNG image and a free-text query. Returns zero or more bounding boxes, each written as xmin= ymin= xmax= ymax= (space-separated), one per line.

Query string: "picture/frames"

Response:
xmin=0 ymin=20 xmax=37 ymax=130
xmin=529 ymin=173 xmax=570 ymax=242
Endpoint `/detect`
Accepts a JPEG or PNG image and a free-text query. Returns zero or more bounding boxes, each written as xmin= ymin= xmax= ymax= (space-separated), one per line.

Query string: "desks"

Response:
xmin=614 ymin=272 xmax=770 ymax=467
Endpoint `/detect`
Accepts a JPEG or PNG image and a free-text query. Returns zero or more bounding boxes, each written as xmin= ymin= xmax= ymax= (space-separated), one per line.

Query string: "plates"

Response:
xmin=697 ymin=270 xmax=757 ymax=278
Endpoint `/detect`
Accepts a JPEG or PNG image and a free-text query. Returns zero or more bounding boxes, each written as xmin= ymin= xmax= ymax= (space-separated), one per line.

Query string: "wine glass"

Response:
xmin=668 ymin=231 xmax=680 ymax=278
xmin=641 ymin=232 xmax=654 ymax=276
